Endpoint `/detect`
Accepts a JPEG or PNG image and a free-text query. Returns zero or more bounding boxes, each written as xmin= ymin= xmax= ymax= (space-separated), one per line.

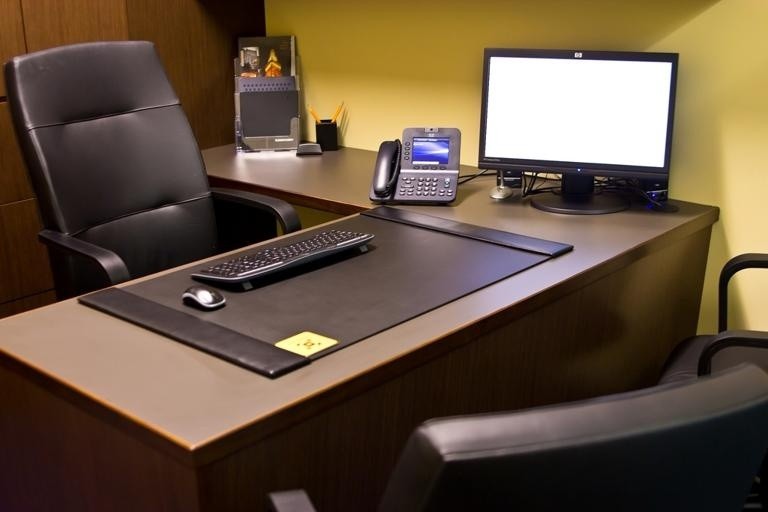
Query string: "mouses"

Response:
xmin=182 ymin=285 xmax=226 ymax=311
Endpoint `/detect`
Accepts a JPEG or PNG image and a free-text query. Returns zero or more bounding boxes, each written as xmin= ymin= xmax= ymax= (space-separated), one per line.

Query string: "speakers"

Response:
xmin=497 ymin=166 xmax=522 ymax=188
xmin=640 ymin=178 xmax=669 ymax=205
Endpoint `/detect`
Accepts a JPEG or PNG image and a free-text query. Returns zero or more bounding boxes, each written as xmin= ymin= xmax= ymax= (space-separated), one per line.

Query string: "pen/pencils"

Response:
xmin=307 ymin=105 xmax=321 ymax=124
xmin=331 ymin=101 xmax=345 ymax=122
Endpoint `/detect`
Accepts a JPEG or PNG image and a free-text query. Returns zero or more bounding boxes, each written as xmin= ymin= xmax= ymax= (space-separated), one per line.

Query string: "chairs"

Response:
xmin=2 ymin=40 xmax=301 ymax=303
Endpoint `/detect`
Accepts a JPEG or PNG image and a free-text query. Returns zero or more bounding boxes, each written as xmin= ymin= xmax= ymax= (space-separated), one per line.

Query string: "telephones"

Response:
xmin=368 ymin=126 xmax=462 ymax=206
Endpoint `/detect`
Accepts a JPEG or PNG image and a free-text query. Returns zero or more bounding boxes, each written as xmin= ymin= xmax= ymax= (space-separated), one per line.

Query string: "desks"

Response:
xmin=1 ymin=139 xmax=719 ymax=512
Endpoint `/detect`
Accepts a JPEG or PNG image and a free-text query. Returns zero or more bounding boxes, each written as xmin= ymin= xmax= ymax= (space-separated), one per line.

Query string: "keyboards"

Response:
xmin=191 ymin=228 xmax=375 ymax=290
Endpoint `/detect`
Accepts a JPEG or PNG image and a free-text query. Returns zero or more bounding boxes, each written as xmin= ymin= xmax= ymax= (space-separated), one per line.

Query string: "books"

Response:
xmin=239 ymin=90 xmax=300 ymax=137
xmin=236 ymin=35 xmax=297 ymax=79
xmin=238 ymin=76 xmax=297 ymax=93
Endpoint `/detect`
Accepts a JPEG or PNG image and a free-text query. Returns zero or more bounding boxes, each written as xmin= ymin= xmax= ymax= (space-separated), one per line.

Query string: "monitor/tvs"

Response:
xmin=478 ymin=48 xmax=678 ymax=215
